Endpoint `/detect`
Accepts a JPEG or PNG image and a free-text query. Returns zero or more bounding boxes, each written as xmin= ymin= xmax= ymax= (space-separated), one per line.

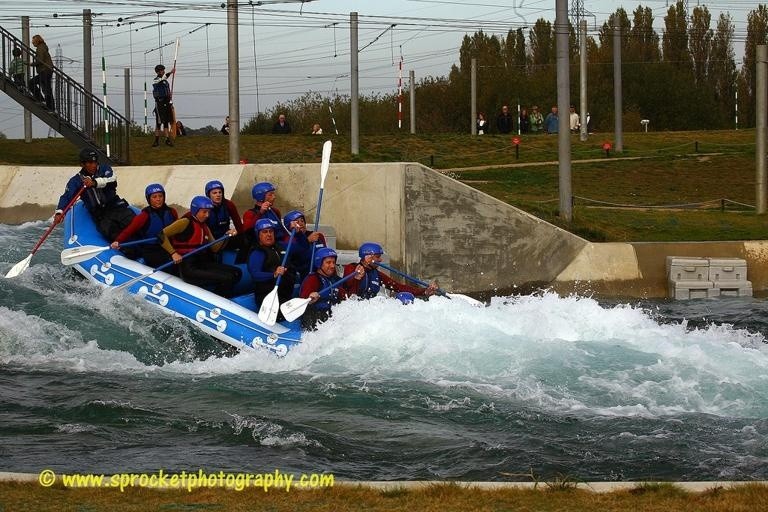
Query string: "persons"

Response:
xmin=496 ymin=105 xmax=514 ymax=134
xmin=311 ymin=123 xmax=323 ymax=135
xmin=519 ymin=103 xmax=596 ymax=133
xmin=476 ymin=112 xmax=489 ymax=136
xmin=52 ymin=148 xmax=146 ymax=260
xmin=272 ymin=114 xmax=292 ymax=135
xmin=8 ymin=48 xmax=27 ymax=95
xmin=152 ymin=65 xmax=177 ymax=148
xmin=157 ymin=181 xmax=439 ymax=331
xmin=221 ymin=117 xmax=230 ymax=135
xmin=176 ymin=121 xmax=187 ymax=137
xmin=27 ymin=35 xmax=56 ymax=113
xmin=111 ymin=184 xmax=179 ymax=268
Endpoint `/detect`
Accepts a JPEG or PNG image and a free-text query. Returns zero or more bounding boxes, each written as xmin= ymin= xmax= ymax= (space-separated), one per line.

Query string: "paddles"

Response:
xmin=371 ymin=259 xmax=485 ymax=308
xmin=308 ymin=141 xmax=332 ymax=270
xmin=258 ymin=227 xmax=296 ymax=327
xmin=280 ymin=270 xmax=360 ymax=322
xmin=111 ymin=233 xmax=232 ymax=292
xmin=61 ymin=238 xmax=157 ymax=264
xmin=5 ymin=184 xmax=89 ymax=279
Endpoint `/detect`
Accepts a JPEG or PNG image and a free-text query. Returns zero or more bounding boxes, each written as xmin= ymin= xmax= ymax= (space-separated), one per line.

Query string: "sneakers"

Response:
xmin=151 ymin=138 xmax=173 ymax=148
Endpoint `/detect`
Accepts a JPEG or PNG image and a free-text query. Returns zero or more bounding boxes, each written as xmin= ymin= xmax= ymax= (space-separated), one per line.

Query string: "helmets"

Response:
xmin=255 ymin=218 xmax=274 ymax=239
xmin=284 ymin=210 xmax=306 ymax=232
xmin=80 ymin=148 xmax=98 ymax=164
xmin=206 ymin=181 xmax=224 ymax=198
xmin=359 ymin=243 xmax=383 ymax=261
xmin=314 ymin=248 xmax=336 ymax=270
xmin=145 ymin=183 xmax=165 ymax=204
xmin=191 ymin=195 xmax=213 ymax=217
xmin=397 ymin=292 xmax=414 ymax=304
xmin=252 ymin=183 xmax=275 ymax=202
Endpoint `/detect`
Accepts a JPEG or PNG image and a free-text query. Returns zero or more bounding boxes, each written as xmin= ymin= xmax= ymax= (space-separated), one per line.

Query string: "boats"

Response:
xmin=64 ymin=193 xmax=346 ymax=359
